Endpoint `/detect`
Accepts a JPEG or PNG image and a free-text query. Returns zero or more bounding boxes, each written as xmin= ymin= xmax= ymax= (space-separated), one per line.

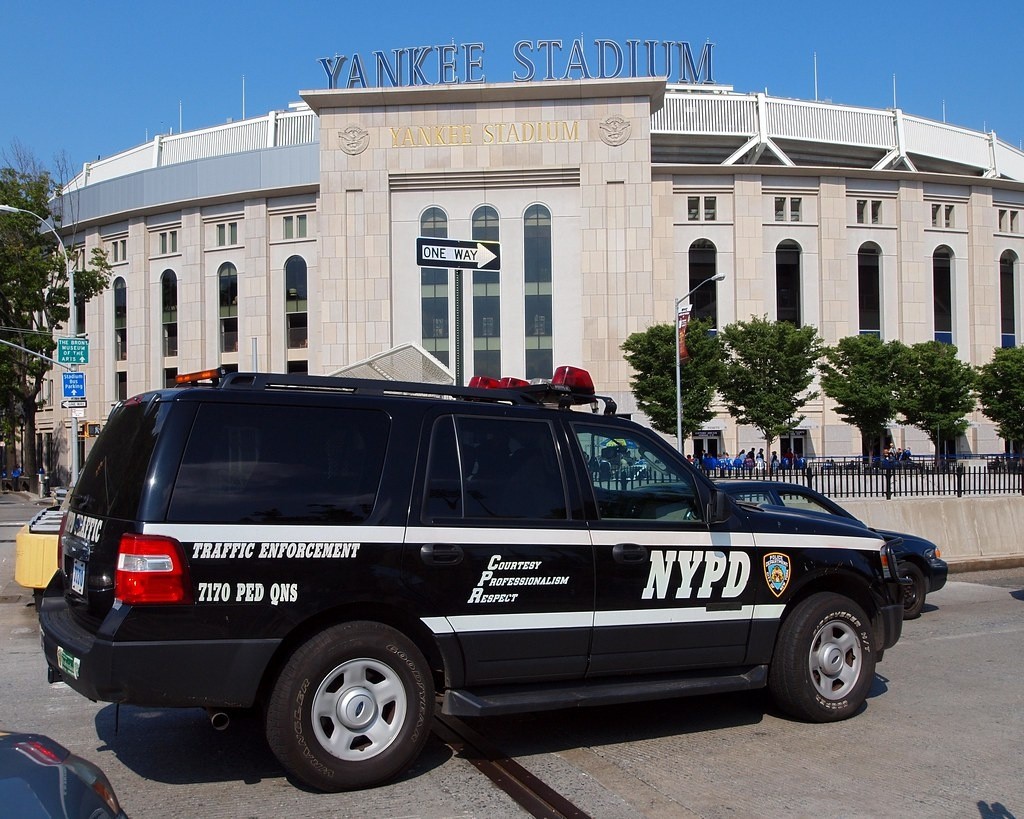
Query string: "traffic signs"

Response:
xmin=60 ymin=401 xmax=87 ymax=409
xmin=415 ymin=237 xmax=503 ymax=273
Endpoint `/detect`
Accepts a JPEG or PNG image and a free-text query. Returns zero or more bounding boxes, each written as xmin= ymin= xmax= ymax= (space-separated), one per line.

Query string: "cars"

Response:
xmin=1 ymin=729 xmax=132 ymax=819
xmin=637 ymin=484 xmax=951 ymax=619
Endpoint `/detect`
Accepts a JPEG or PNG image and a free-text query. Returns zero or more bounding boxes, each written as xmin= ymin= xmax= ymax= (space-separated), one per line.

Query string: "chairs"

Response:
xmin=182 ymin=458 xmax=568 ymax=523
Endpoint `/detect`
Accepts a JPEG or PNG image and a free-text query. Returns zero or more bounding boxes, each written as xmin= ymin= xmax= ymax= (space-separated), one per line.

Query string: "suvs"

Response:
xmin=35 ymin=364 xmax=906 ymax=794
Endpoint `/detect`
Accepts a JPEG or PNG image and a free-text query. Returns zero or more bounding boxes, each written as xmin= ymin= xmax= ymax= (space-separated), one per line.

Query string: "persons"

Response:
xmin=884 ymin=443 xmax=911 ymax=461
xmin=733 ymin=448 xmax=755 ymax=469
xmin=701 ymin=450 xmax=734 ymax=470
xmin=771 ymin=451 xmax=776 ymax=463
xmin=11 ymin=464 xmax=21 ymax=474
xmin=687 ymin=454 xmax=699 ymax=466
xmin=583 ymin=451 xmax=646 ymax=474
xmin=782 ymin=447 xmax=793 ymax=461
xmin=756 ymin=449 xmax=763 ymax=459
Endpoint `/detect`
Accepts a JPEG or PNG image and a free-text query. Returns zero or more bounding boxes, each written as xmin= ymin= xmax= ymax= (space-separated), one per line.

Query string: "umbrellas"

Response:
xmin=596 ymin=438 xmax=638 ymax=449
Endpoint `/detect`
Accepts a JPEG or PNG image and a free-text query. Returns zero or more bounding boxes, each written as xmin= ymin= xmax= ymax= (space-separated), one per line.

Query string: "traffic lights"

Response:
xmin=85 ymin=422 xmax=101 ymax=436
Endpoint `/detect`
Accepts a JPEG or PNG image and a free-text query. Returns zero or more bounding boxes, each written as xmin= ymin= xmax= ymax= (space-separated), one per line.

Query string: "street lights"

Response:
xmin=674 ymin=273 xmax=728 ymax=452
xmin=0 ymin=205 xmax=80 ymax=487
